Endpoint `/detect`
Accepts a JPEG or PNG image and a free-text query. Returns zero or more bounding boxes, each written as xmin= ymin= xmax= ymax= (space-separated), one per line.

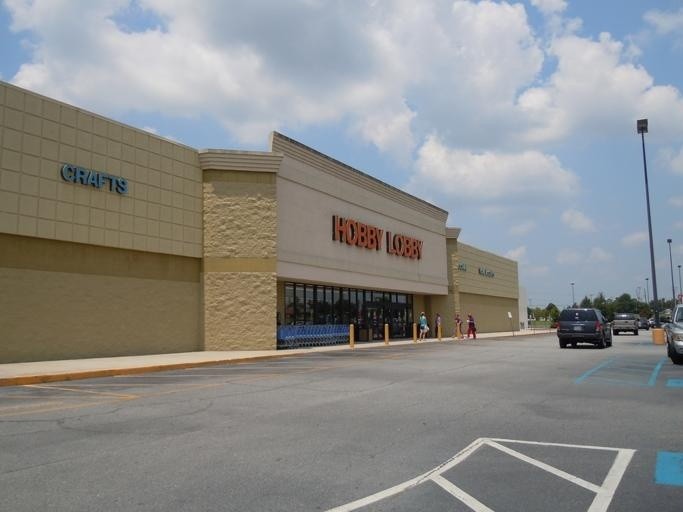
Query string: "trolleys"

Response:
xmin=277 ymin=325 xmax=352 ymax=349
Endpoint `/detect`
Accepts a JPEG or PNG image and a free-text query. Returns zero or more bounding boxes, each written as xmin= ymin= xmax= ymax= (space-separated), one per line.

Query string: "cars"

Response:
xmin=663 ymin=303 xmax=682 ymax=364
xmin=557 ymin=305 xmax=655 ymax=350
xmin=352 ymin=306 xmax=410 ymax=337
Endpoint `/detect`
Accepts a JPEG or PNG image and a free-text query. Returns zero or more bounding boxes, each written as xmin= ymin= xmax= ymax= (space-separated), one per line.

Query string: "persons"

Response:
xmin=434 ymin=312 xmax=441 ymax=337
xmin=466 ymin=313 xmax=476 ymax=339
xmin=451 ymin=314 xmax=464 ymax=338
xmin=419 ymin=312 xmax=427 ymax=341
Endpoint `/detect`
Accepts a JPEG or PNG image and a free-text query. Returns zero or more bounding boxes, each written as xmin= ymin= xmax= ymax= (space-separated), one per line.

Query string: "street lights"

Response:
xmin=571 ymin=282 xmax=575 ymax=307
xmin=636 ymin=118 xmax=683 ymax=343
